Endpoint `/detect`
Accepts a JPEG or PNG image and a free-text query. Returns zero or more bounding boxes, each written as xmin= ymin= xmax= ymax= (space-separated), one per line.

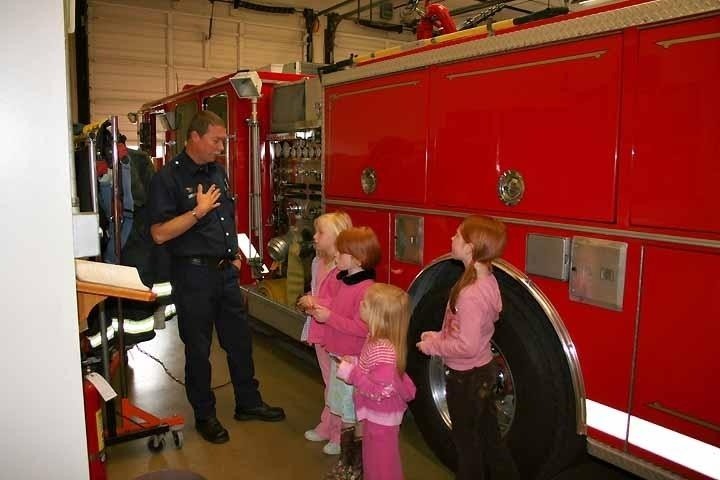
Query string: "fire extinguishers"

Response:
xmin=84 ymin=358 xmax=106 ymax=479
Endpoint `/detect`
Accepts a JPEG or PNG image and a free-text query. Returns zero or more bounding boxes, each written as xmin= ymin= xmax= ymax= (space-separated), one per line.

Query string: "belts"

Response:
xmin=192 ymin=257 xmax=227 ymax=269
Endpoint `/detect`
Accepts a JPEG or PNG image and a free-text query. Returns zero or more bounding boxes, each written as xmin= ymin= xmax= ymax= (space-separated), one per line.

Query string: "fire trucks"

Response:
xmin=125 ymin=0 xmax=719 ymax=480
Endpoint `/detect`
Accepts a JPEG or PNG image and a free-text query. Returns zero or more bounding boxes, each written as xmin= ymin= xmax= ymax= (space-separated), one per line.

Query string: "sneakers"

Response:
xmin=304 ymin=429 xmax=327 ymax=442
xmin=322 ymin=442 xmax=341 ymax=455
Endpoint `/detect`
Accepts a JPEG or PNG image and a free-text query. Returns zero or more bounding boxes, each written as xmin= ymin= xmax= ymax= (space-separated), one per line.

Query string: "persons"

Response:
xmin=145 ymin=110 xmax=286 ymax=444
xmin=95 ymin=133 xmax=156 ymax=289
xmin=295 ymin=212 xmax=417 ymax=480
xmin=415 ymin=215 xmax=507 ymax=480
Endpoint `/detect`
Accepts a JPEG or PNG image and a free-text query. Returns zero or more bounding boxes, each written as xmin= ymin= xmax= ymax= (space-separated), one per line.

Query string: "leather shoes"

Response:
xmin=233 ymin=402 xmax=286 ymax=421
xmin=195 ymin=416 xmax=229 ymax=444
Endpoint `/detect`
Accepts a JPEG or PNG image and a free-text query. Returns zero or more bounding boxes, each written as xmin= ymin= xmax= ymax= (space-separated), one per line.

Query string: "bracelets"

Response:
xmin=191 ymin=210 xmax=201 ymax=222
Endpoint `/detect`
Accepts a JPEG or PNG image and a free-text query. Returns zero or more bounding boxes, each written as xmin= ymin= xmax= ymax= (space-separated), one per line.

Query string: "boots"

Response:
xmin=326 ymin=427 xmax=363 ymax=480
